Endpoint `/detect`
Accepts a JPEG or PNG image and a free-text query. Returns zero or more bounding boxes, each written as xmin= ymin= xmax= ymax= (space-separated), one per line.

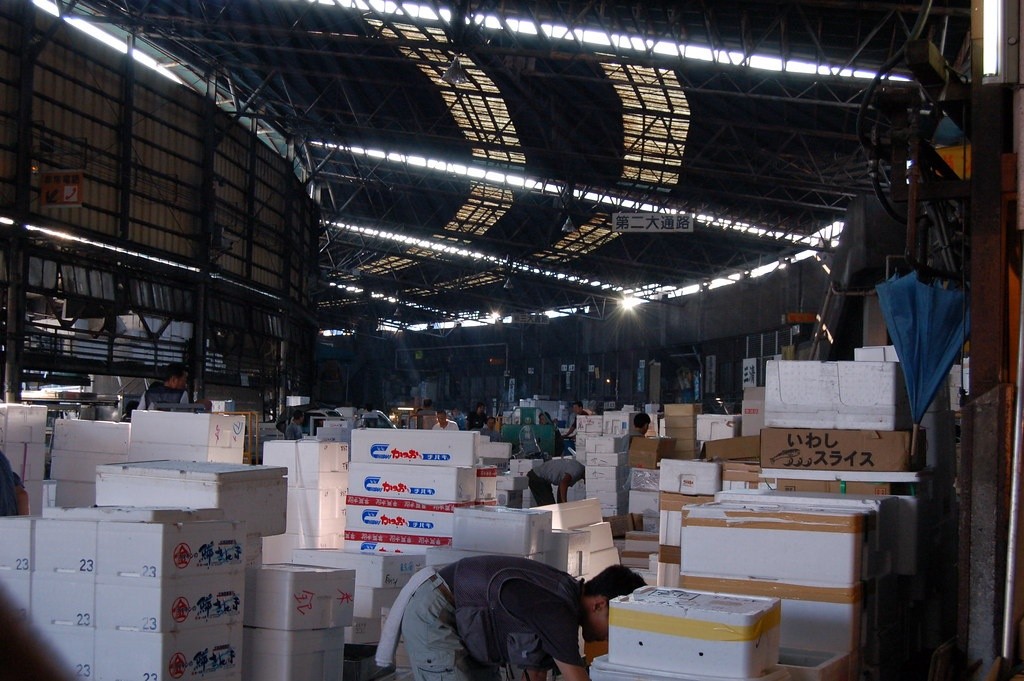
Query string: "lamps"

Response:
xmin=981 ymin=0 xmax=1018 ymax=84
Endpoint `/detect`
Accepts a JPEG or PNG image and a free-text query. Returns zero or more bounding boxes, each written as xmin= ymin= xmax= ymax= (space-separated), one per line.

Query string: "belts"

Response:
xmin=429 ymin=574 xmax=456 ymax=610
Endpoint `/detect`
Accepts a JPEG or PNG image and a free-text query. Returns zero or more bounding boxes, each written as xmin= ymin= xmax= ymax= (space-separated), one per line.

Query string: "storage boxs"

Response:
xmin=0 ymin=289 xmax=925 ymax=681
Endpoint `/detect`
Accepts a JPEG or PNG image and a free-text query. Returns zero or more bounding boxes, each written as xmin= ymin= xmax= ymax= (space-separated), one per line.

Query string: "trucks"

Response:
xmin=243 ymin=407 xmax=398 ymax=465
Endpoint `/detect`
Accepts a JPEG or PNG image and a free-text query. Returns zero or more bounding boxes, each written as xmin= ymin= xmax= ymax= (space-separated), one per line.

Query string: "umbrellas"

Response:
xmin=877 ymin=267 xmax=968 ymax=456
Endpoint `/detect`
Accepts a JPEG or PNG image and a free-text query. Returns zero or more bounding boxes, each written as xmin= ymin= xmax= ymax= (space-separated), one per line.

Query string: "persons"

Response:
xmin=565 ymin=401 xmax=589 ymax=439
xmin=315 ymin=419 xmax=323 ymax=426
xmin=418 ymin=399 xmax=435 ymax=414
xmin=480 ymin=416 xmax=500 ymax=442
xmin=285 ymin=409 xmax=304 ymax=440
xmin=466 ymin=402 xmax=488 ymax=431
xmin=452 ymin=408 xmax=465 ymax=429
xmin=136 ymin=362 xmax=212 ymax=412
xmin=401 ymin=556 xmax=647 ymax=681
xmin=620 ymin=413 xmax=650 ymax=451
xmin=539 ymin=412 xmax=565 ymax=457
xmin=527 ymin=458 xmax=588 ymax=504
xmin=431 ymin=410 xmax=459 ymax=431
xmin=0 ymin=450 xmax=30 ymax=515
